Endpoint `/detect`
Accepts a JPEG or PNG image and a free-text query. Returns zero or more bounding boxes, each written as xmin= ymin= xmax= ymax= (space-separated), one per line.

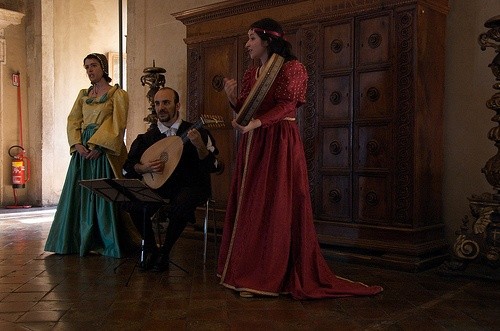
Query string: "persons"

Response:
xmin=45 ymin=53 xmax=130 ymax=259
xmin=121 ymin=87 xmax=224 ymax=272
xmin=215 ymin=17 xmax=384 ymax=299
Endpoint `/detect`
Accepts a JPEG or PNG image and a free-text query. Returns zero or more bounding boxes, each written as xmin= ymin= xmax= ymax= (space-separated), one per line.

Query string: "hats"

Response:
xmin=93 ymin=53 xmax=109 ymax=75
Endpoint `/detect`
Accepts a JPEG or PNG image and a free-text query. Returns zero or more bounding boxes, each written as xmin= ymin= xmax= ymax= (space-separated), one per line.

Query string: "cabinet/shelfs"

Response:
xmin=170 ymin=0 xmax=448 ymax=273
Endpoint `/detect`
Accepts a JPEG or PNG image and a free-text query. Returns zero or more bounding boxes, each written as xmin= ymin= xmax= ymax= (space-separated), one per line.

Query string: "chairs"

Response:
xmin=148 ymin=169 xmax=218 ymax=268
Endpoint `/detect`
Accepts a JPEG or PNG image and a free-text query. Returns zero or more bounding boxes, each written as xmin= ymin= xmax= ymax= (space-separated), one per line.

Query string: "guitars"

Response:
xmin=140 ymin=114 xmax=226 ymax=190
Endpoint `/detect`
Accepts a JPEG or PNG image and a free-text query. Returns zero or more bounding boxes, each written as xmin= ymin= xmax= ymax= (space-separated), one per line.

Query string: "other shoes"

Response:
xmin=151 ymin=249 xmax=170 ymax=272
xmin=136 ymin=246 xmax=159 ymax=271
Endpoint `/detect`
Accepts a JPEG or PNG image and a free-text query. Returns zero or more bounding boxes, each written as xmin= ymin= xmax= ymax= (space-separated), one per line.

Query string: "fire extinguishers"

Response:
xmin=7 ymin=145 xmax=31 ymax=190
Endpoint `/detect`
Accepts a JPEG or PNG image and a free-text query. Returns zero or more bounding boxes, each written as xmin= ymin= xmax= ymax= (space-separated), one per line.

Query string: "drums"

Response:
xmin=235 ymin=53 xmax=285 ymax=125
